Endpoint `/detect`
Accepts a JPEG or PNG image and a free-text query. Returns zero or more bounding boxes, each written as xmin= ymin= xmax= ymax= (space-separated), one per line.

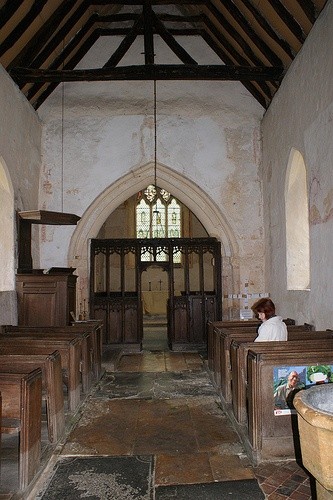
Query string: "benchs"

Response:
xmin=205 ymin=317 xmax=333 ymax=463
xmin=0 ymin=320 xmax=105 ymax=490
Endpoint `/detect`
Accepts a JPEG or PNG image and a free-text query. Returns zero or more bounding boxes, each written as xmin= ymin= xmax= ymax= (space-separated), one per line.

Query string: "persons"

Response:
xmin=274 ymin=370 xmax=302 ymax=410
xmin=251 ymin=297 xmax=289 ymax=341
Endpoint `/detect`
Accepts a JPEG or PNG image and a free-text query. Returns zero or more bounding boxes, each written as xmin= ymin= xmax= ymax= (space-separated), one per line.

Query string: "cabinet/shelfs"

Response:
xmin=15 ymin=273 xmax=80 ymax=326
